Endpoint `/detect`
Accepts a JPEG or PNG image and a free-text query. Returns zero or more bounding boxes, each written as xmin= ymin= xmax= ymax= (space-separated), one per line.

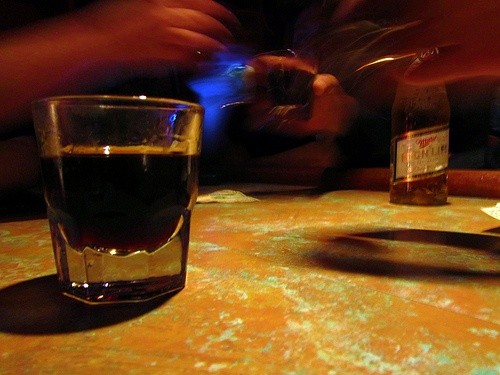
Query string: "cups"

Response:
xmin=29 ymin=92 xmax=205 ymax=306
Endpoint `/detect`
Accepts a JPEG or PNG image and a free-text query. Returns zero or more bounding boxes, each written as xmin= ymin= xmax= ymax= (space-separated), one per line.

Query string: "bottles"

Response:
xmin=388 ymin=45 xmax=453 ymax=210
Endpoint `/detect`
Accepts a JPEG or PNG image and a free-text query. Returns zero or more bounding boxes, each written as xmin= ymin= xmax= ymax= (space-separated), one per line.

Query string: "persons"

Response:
xmin=0 ymin=0 xmax=500 ymax=215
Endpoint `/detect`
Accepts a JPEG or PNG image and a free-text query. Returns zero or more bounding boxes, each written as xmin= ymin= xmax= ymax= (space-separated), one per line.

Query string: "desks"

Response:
xmin=2 ymin=180 xmax=499 ymax=375
